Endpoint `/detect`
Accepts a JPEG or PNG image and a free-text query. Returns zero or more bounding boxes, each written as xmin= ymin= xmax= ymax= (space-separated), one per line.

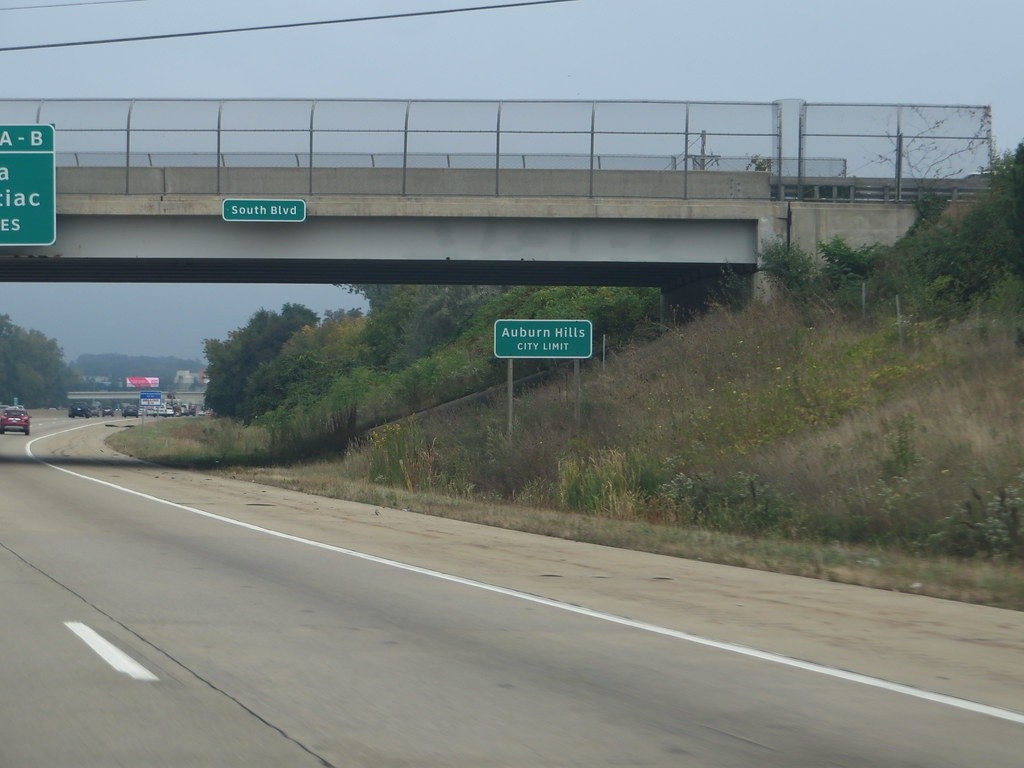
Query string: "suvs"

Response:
xmin=69 ymin=401 xmax=90 ymax=419
xmin=0 ymin=405 xmax=33 ymax=435
xmin=121 ymin=404 xmax=139 ymax=418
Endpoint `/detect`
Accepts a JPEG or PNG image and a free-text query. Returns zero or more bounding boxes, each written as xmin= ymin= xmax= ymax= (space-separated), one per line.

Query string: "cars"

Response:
xmin=102 ymin=406 xmax=114 ymax=418
xmin=88 ymin=404 xmax=101 ymax=417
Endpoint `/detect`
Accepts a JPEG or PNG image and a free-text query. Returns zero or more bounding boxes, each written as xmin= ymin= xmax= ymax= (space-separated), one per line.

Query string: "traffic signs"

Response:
xmin=0 ymin=122 xmax=58 ymax=247
xmin=494 ymin=318 xmax=593 ymax=359
xmin=221 ymin=198 xmax=307 ymax=223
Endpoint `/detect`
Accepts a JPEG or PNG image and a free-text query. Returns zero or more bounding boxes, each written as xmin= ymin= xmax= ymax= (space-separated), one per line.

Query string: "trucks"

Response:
xmin=138 ymin=391 xmax=164 ymax=417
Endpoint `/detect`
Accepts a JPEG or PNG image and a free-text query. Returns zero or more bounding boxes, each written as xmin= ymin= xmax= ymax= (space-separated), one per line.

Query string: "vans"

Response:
xmin=163 ymin=399 xmax=213 ymax=417
